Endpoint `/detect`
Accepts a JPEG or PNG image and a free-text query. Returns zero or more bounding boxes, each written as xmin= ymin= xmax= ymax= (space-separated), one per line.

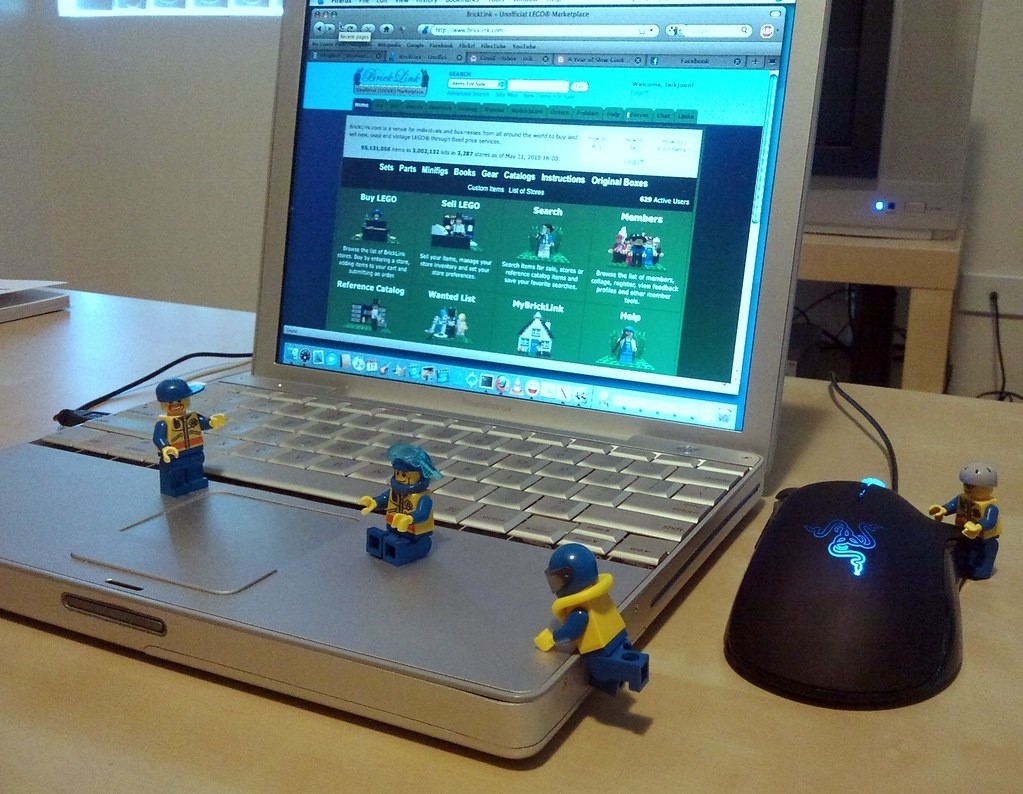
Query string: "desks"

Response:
xmin=797 ymin=234 xmax=966 ymax=394
xmin=0 ymin=285 xmax=1023 ymax=794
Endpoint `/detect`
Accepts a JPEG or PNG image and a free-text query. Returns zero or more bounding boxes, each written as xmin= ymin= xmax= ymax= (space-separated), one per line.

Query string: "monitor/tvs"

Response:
xmin=801 ymin=0 xmax=985 ymax=241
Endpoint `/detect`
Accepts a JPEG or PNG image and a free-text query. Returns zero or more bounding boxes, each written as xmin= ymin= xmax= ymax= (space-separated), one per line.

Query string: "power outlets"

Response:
xmin=958 ymin=273 xmax=1023 ymax=316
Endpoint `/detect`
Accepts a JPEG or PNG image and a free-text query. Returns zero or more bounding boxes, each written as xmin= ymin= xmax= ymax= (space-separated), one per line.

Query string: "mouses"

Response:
xmin=727 ymin=476 xmax=969 ymax=710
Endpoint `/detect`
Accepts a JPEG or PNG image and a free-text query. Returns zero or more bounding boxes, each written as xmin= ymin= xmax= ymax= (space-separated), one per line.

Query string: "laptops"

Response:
xmin=0 ymin=0 xmax=828 ymax=763
